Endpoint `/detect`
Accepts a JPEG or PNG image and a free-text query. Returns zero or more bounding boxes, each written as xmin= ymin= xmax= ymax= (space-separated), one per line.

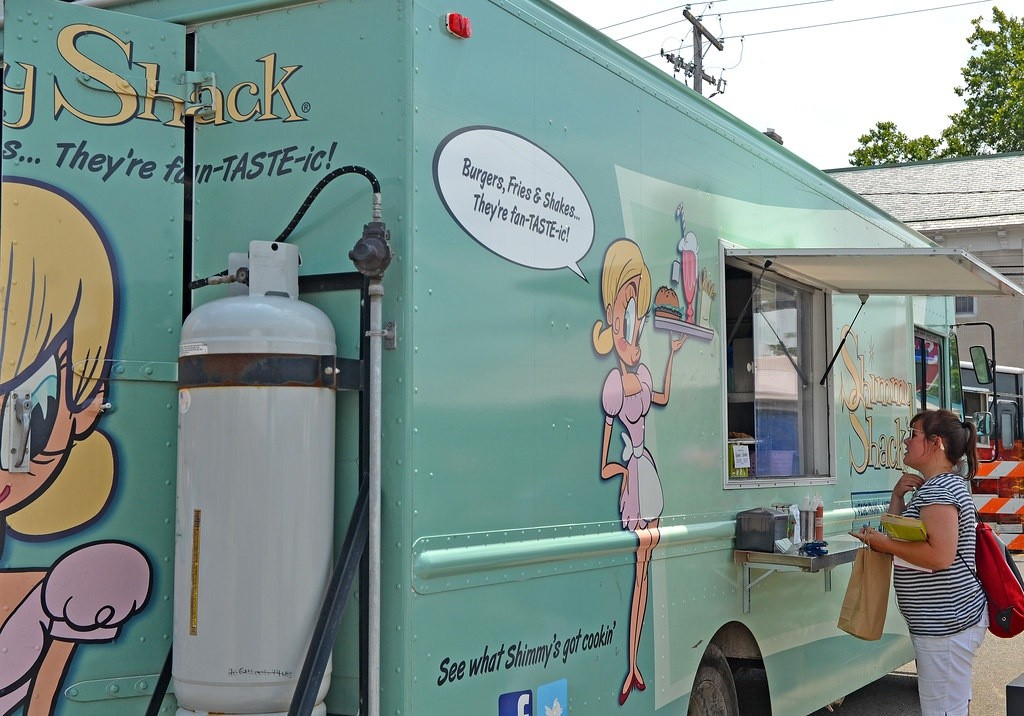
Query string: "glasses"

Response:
xmin=909 ymin=428 xmax=945 ymax=451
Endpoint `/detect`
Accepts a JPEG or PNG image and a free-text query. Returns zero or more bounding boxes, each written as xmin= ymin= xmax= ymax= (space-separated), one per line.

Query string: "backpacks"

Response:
xmin=957 ymin=504 xmax=1024 ymax=638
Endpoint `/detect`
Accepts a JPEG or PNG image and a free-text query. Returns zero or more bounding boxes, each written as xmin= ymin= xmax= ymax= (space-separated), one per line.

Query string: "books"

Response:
xmin=880 ymin=513 xmax=934 ymax=573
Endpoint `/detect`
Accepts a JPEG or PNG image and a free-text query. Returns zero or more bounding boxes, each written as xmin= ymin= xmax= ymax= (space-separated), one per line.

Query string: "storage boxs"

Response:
xmin=747 ymin=449 xmax=798 ymax=478
xmin=735 ymin=506 xmax=791 ymax=554
xmin=728 ymin=443 xmax=749 ymax=478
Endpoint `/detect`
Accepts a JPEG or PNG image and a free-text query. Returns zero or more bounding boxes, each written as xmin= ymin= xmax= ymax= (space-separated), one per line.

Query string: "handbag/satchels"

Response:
xmin=837 ymin=531 xmax=893 ymax=641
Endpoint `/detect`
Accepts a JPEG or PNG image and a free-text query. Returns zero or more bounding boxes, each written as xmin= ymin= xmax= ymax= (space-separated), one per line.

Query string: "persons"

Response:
xmin=850 ymin=409 xmax=989 ymax=716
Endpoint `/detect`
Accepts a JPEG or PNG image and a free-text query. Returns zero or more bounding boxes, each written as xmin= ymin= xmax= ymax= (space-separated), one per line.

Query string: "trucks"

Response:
xmin=0 ymin=0 xmax=999 ymax=716
xmin=914 ymin=355 xmax=1024 ymax=553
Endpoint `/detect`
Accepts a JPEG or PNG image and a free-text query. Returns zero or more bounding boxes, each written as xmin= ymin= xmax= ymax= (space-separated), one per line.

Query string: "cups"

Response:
xmin=798 ymin=509 xmax=818 ymax=543
